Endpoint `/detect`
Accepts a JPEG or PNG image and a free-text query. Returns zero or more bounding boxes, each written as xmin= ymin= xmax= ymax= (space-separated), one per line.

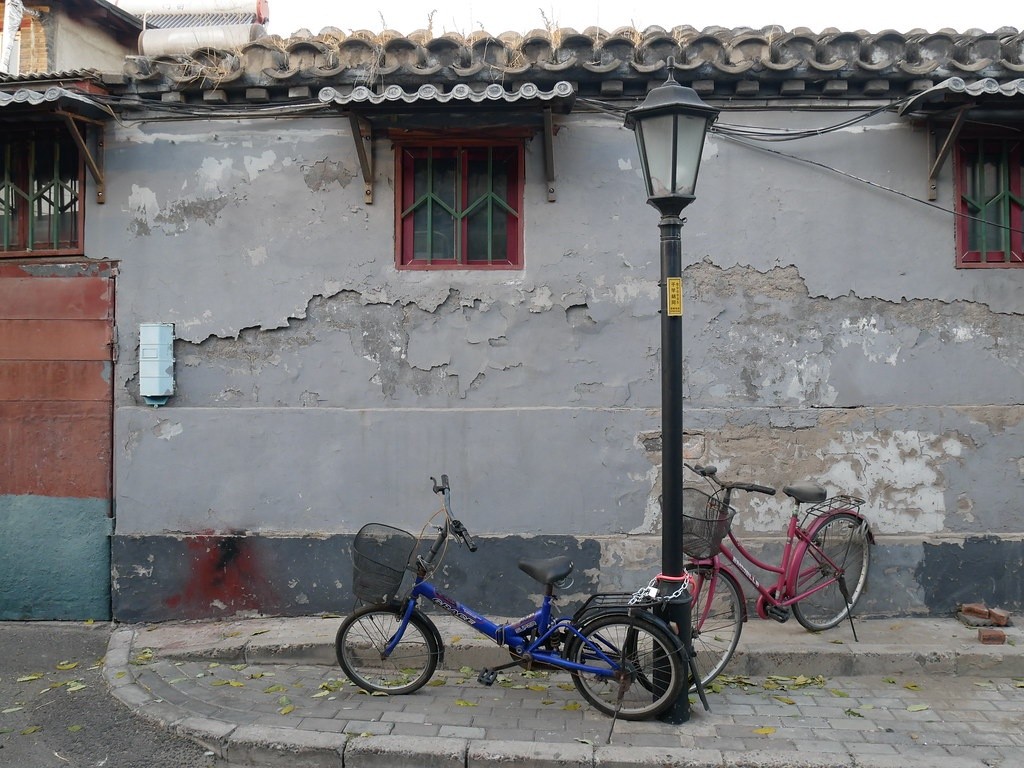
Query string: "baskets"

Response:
xmin=658 ymin=487 xmax=737 ymax=560
xmin=350 ymin=522 xmax=418 ymax=607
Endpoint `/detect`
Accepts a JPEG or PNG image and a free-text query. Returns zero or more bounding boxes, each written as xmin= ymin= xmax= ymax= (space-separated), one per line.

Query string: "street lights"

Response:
xmin=619 ymin=54 xmax=722 ymax=726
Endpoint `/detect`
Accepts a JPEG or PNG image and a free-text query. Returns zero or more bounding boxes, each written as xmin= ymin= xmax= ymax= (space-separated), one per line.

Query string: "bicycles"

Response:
xmin=626 ymin=460 xmax=877 ymax=696
xmin=333 ymin=472 xmax=693 ymax=747
